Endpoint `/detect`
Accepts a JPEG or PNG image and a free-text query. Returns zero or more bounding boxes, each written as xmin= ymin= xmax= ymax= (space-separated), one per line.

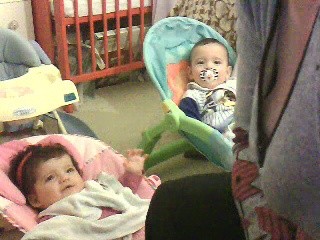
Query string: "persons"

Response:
xmin=9 ymin=143 xmax=152 ymax=240
xmin=145 ymin=0 xmax=320 ymax=240
xmin=180 ymin=38 xmax=237 ymax=146
